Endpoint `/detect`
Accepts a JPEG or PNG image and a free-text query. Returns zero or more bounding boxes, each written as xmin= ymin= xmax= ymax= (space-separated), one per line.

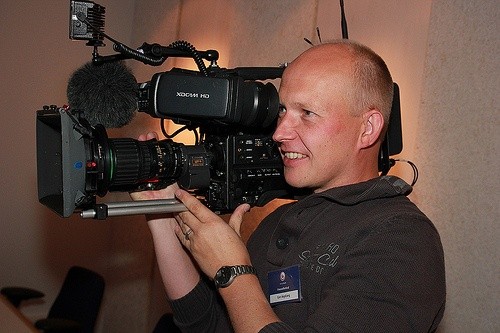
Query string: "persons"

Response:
xmin=128 ymin=40 xmax=446 ymax=333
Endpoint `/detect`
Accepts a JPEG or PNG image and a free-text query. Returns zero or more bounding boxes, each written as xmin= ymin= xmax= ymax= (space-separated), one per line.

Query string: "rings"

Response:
xmin=187 ymin=230 xmax=193 ymax=235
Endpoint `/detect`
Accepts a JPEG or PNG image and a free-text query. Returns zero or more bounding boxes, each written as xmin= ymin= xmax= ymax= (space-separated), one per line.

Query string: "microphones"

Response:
xmin=67 ymin=56 xmax=142 ymax=127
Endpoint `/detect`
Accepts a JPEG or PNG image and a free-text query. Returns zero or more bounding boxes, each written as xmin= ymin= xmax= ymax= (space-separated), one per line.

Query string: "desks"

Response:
xmin=0 ymin=291 xmax=38 ymax=333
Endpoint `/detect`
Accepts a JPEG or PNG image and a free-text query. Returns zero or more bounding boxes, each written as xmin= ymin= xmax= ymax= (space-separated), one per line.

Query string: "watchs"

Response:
xmin=215 ymin=265 xmax=259 ymax=289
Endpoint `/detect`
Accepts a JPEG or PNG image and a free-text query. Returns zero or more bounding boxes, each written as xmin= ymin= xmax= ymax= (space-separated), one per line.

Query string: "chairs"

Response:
xmin=0 ymin=266 xmax=108 ymax=333
